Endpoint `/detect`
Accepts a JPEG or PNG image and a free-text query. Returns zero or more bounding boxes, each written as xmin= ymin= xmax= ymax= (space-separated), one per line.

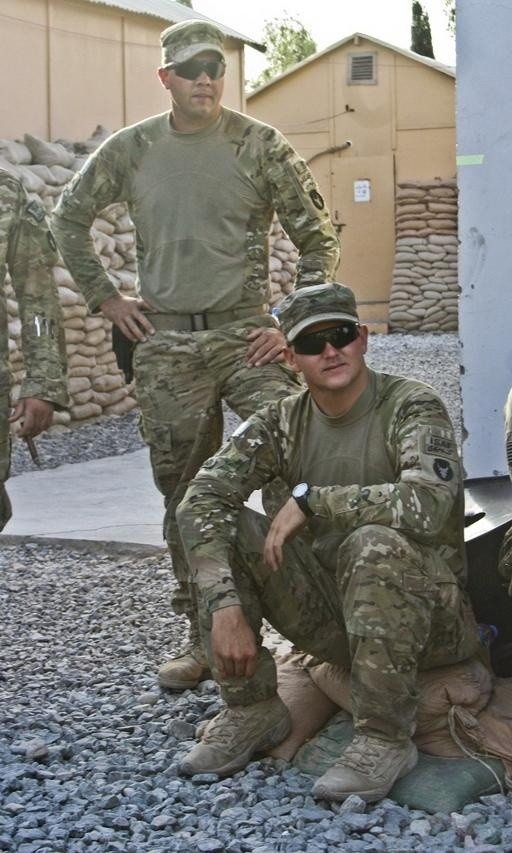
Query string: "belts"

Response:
xmin=146 ymin=306 xmax=261 ymax=331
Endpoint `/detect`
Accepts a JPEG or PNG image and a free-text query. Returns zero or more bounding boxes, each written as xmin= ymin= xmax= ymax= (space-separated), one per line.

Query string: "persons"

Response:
xmin=0 ymin=168 xmax=72 ymax=535
xmin=178 ymin=282 xmax=477 ymax=803
xmin=500 ymin=386 xmax=511 ymax=629
xmin=42 ymin=21 xmax=344 ymax=691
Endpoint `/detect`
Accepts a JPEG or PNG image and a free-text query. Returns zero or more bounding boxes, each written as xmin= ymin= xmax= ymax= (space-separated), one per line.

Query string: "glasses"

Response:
xmin=175 ymin=59 xmax=226 ymax=80
xmin=294 ymin=323 xmax=357 ymax=354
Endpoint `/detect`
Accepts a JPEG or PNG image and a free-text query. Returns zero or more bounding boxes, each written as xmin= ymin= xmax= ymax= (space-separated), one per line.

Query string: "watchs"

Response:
xmin=292 ymin=483 xmax=315 ymax=518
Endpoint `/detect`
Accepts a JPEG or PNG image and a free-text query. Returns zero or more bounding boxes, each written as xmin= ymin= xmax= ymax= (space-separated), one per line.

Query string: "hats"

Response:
xmin=160 ymin=19 xmax=225 ymax=68
xmin=277 ymin=283 xmax=359 ymax=343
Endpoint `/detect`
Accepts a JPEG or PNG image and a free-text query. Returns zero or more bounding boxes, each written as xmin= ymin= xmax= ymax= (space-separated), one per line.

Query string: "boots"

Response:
xmin=158 ymin=611 xmax=207 ymax=690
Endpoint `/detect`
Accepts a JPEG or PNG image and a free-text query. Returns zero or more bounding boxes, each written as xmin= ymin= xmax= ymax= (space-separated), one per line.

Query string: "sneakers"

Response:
xmin=310 ymin=736 xmax=417 ymax=804
xmin=180 ymin=694 xmax=290 ymax=776
